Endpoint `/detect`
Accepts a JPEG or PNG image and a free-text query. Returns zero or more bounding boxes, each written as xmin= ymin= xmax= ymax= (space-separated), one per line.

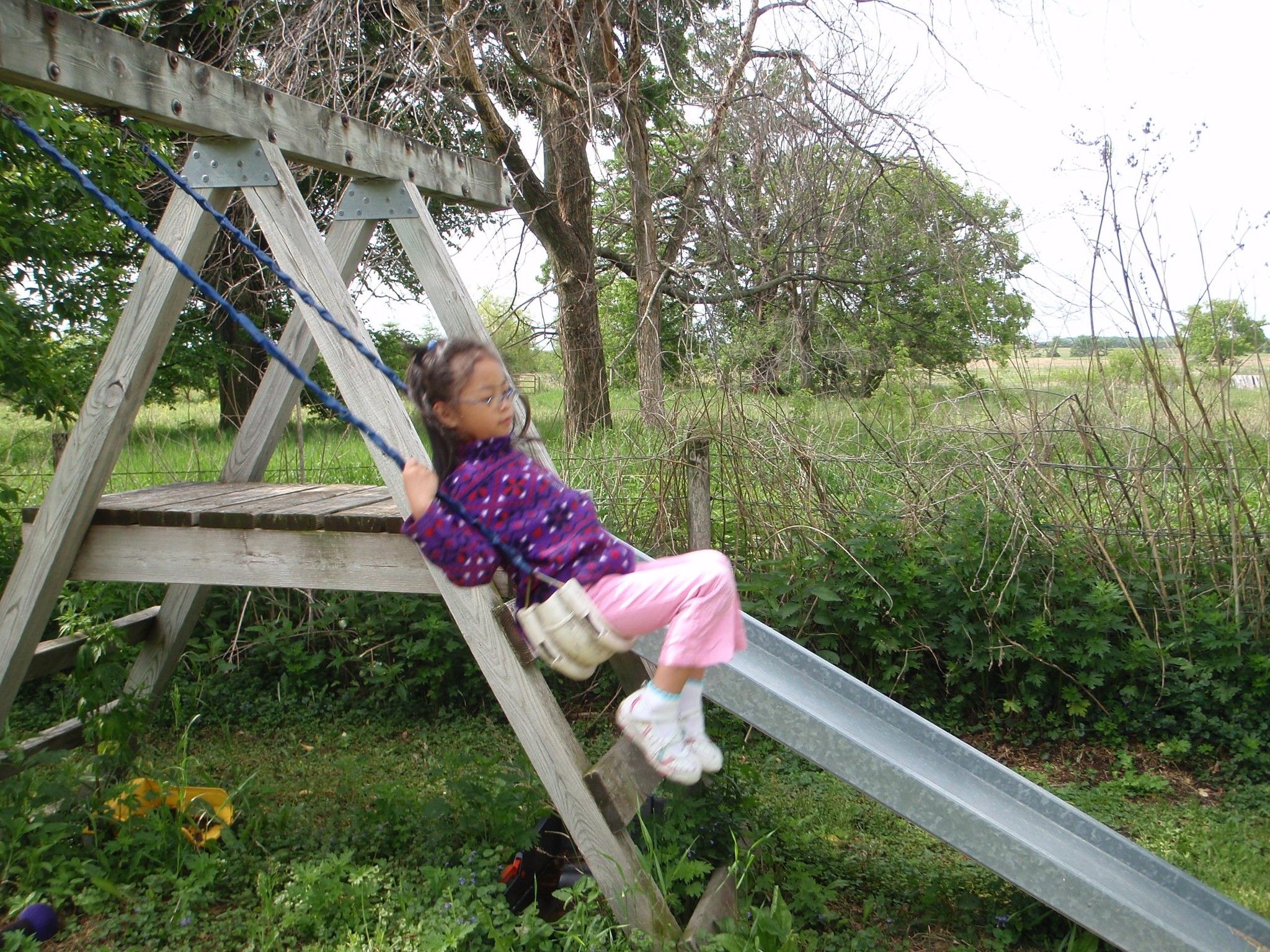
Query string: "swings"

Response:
xmin=0 ymin=96 xmax=639 ymax=682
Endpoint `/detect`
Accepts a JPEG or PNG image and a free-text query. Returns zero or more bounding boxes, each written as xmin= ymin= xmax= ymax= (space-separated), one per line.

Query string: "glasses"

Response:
xmin=449 ymin=387 xmax=520 ymax=409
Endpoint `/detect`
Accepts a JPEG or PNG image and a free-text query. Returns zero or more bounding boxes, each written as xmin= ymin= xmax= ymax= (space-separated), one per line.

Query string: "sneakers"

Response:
xmin=676 ymin=703 xmax=724 ymax=774
xmin=614 ymin=679 xmax=704 ymax=785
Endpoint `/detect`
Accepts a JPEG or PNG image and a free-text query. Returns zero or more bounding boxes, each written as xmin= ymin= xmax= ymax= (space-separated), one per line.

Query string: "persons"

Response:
xmin=398 ymin=338 xmax=746 ymax=787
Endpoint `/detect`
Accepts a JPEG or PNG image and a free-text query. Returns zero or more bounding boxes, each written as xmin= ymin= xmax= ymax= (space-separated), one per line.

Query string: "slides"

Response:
xmin=594 ymin=526 xmax=1270 ymax=952
